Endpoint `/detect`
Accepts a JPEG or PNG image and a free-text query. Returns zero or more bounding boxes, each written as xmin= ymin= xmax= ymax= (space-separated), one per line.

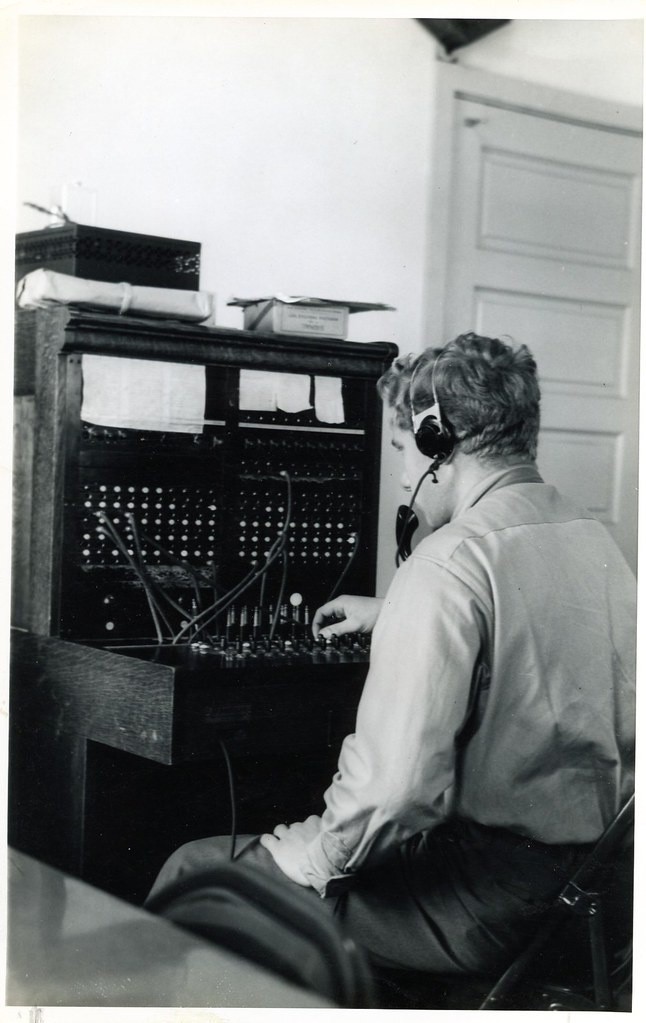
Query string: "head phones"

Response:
xmin=409 ymin=352 xmax=457 ymax=459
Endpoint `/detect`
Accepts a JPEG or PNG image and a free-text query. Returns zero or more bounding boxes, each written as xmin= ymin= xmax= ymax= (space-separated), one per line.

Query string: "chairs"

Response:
xmin=139 ymin=793 xmax=632 ymax=1013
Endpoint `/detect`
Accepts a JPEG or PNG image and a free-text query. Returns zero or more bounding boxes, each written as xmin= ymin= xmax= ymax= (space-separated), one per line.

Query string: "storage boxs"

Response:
xmin=243 ymin=299 xmax=348 ymax=340
xmin=14 ymin=225 xmax=201 ymax=314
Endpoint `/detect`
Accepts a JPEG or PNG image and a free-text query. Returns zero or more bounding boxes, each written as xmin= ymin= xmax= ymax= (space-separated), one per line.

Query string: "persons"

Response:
xmin=145 ymin=333 xmax=637 ymax=973
